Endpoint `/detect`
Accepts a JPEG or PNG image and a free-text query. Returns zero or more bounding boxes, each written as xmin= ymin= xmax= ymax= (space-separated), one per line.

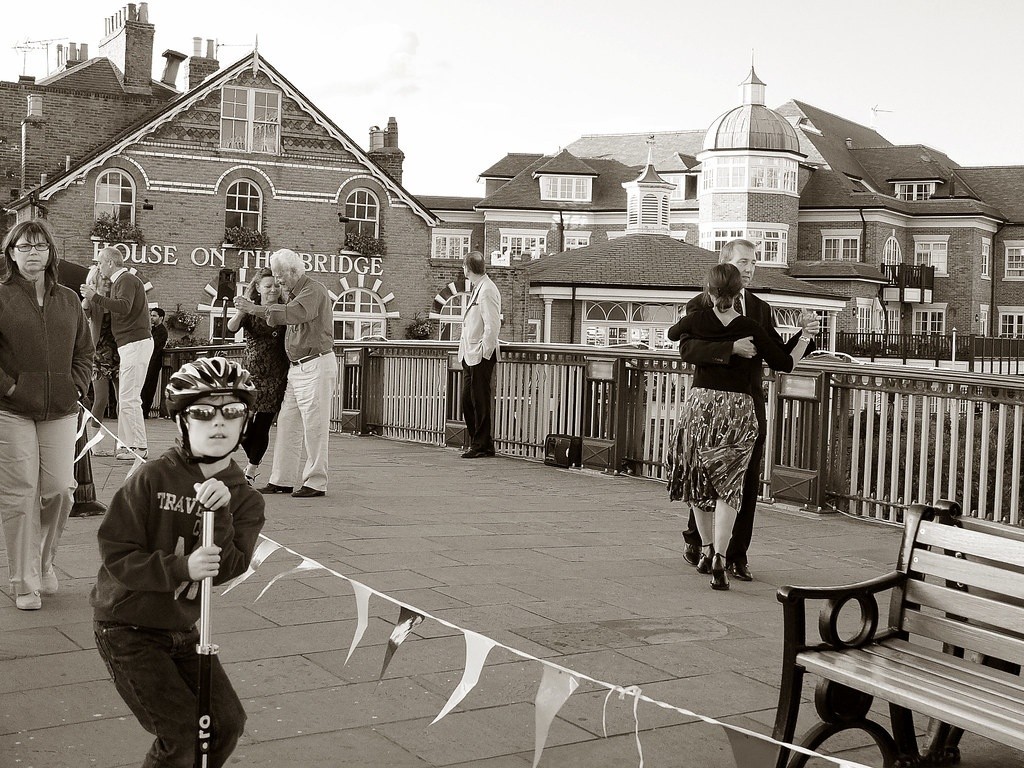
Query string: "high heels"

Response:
xmin=89 ymin=447 xmax=108 ymax=456
xmin=696 ymin=543 xmax=715 ymax=574
xmin=710 ymin=553 xmax=730 ymax=590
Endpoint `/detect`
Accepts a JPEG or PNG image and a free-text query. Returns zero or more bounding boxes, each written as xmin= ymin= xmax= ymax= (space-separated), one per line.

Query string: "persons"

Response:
xmin=683 ymin=238 xmax=824 ymax=581
xmin=0 ymin=222 xmax=95 ymax=608
xmin=78 ymin=245 xmax=339 ymax=497
xmin=456 ymin=252 xmax=502 ymax=458
xmin=667 ymin=263 xmax=817 ymax=590
xmin=86 ymin=356 xmax=272 ymax=768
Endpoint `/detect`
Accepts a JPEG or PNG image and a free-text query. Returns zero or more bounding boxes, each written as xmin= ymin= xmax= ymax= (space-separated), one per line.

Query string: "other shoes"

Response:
xmin=243 ymin=460 xmax=262 ymax=487
xmin=41 ymin=564 xmax=58 ymax=595
xmin=16 ymin=589 xmax=41 ymax=610
xmin=144 ymin=415 xmax=150 ymax=419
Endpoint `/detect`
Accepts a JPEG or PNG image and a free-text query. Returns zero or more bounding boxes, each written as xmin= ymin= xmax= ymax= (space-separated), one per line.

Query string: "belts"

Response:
xmin=289 ymin=350 xmax=334 ymax=366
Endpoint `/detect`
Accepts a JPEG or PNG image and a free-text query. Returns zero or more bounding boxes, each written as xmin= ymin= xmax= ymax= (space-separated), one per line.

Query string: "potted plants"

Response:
xmin=89 ymin=206 xmax=146 ymax=249
xmin=165 ymin=303 xmax=204 ymax=334
xmin=224 ymin=226 xmax=271 ymax=253
xmin=405 ymin=312 xmax=437 ymax=339
xmin=344 ymin=231 xmax=387 ymax=257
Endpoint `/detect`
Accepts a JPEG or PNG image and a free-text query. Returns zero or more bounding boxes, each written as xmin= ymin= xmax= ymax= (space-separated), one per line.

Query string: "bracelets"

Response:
xmin=799 ymin=336 xmax=810 ymax=345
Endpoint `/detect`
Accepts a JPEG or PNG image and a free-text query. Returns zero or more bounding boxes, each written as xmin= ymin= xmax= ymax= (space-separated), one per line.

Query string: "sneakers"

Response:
xmin=461 ymin=448 xmax=495 ymax=458
xmin=115 ymin=448 xmax=148 ymax=459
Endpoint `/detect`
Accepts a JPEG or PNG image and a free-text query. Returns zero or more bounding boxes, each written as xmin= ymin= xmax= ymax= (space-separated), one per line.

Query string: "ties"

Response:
xmin=733 ymin=292 xmax=743 ymax=316
xmin=467 ymin=285 xmax=476 ymax=306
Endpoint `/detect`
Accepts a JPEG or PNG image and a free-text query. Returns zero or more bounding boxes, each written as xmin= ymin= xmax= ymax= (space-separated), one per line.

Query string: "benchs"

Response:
xmin=760 ymin=499 xmax=1024 ymax=768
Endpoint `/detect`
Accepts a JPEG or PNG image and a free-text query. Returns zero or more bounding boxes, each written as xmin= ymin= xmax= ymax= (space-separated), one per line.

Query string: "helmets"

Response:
xmin=165 ymin=356 xmax=258 ymax=424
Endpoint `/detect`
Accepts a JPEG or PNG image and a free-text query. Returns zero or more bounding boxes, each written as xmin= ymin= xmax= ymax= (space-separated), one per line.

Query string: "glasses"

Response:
xmin=180 ymin=401 xmax=250 ymax=421
xmin=462 ymin=265 xmax=465 ymax=268
xmin=14 ymin=243 xmax=50 ymax=252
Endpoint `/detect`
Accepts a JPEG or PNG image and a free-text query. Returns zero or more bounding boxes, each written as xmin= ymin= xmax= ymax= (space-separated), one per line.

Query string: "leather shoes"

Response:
xmin=291 ymin=485 xmax=326 ymax=498
xmin=257 ymin=483 xmax=294 ymax=494
xmin=683 ymin=541 xmax=702 ymax=566
xmin=725 ymin=557 xmax=753 ymax=581
